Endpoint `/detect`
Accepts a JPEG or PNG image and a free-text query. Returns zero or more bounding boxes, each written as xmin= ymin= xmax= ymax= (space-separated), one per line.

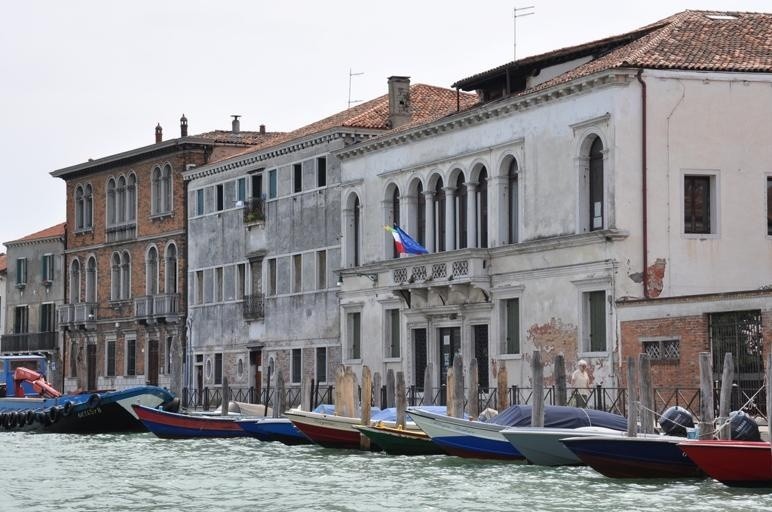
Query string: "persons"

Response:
xmin=571 ymin=359 xmax=592 ymax=409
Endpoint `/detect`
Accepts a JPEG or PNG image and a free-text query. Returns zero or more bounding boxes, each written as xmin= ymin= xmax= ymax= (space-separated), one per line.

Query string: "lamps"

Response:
xmin=89 ymin=304 xmax=115 ymax=323
xmin=235 ymin=195 xmax=265 ymax=208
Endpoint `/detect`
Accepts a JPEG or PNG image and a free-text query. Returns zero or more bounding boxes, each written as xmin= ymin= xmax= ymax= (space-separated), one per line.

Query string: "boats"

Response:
xmin=349 ymin=420 xmax=436 ymax=455
xmin=556 ymin=413 xmax=763 ymax=482
xmin=0 ymin=354 xmax=185 ymax=435
xmin=403 ymin=404 xmax=525 ymax=461
xmin=228 ymin=414 xmax=308 ymax=445
xmin=128 ymin=399 xmax=252 ymax=441
xmin=282 ymin=407 xmax=422 ymax=453
xmin=673 ymin=437 xmax=772 ymax=489
xmin=491 ymin=406 xmax=696 ymax=468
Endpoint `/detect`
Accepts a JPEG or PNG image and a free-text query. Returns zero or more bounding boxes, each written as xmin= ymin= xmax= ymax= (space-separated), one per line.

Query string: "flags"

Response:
xmin=386 ymin=226 xmax=421 ymax=257
xmin=396 ymin=227 xmax=428 ymax=253
